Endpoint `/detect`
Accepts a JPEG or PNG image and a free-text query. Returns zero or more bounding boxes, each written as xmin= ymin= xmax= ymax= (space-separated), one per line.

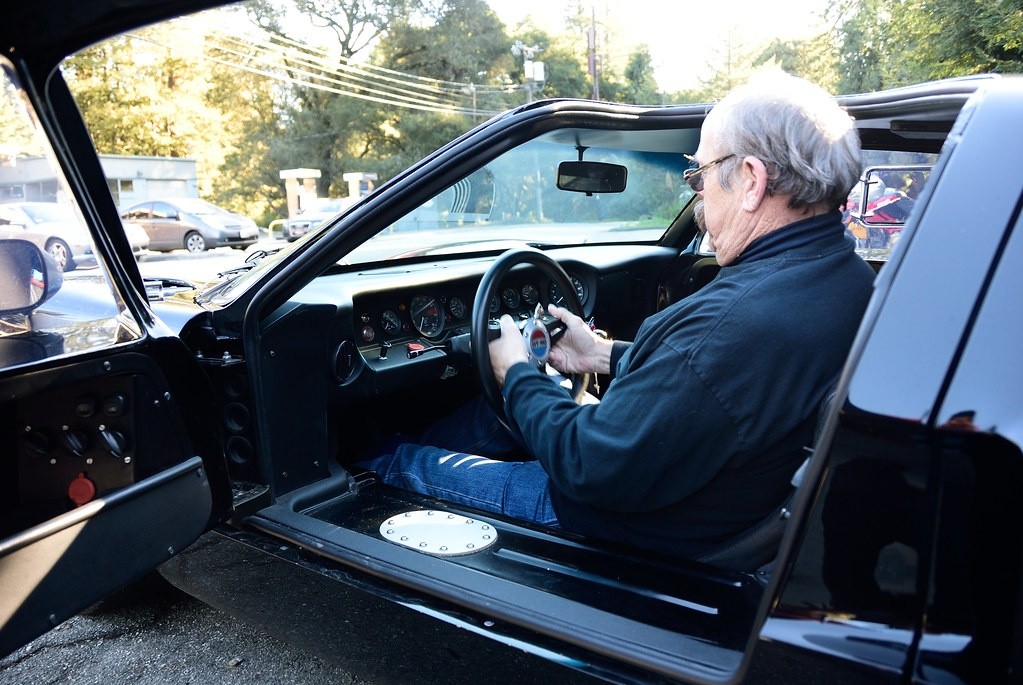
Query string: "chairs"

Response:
xmin=694 ymin=379 xmax=837 ymax=572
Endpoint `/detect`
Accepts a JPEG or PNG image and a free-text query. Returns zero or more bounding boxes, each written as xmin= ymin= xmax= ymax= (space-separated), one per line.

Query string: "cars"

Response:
xmin=0 ymin=1 xmax=1022 ymax=685
xmin=0 ymin=201 xmax=150 ymax=272
xmin=120 ymin=195 xmax=260 ymax=253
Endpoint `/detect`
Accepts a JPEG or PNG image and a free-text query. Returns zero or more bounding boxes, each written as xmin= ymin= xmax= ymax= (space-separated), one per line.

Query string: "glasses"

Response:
xmin=684 ymin=153 xmax=737 ymax=192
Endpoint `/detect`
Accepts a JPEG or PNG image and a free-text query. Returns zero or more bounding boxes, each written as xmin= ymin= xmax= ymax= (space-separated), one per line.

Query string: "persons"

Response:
xmin=881 ymin=173 xmax=905 ymax=195
xmin=359 ymin=75 xmax=875 ymax=560
